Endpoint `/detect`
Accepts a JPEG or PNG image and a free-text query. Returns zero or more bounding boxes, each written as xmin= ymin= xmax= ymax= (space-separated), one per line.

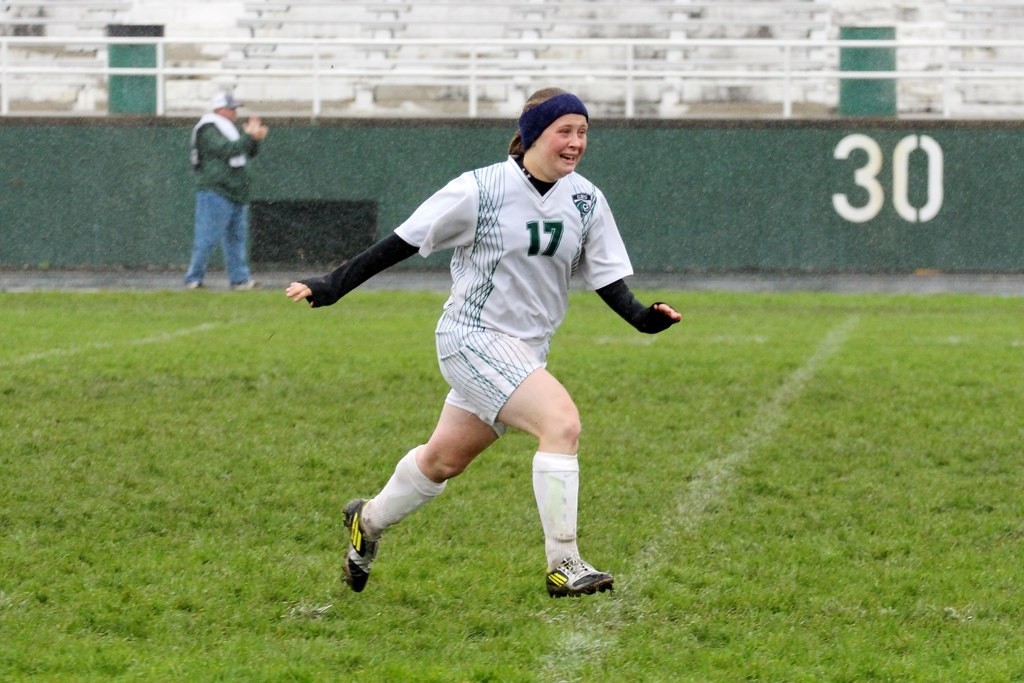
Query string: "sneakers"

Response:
xmin=545 ymin=555 xmax=614 ymax=598
xmin=343 ymin=499 xmax=383 ymax=592
xmin=232 ymin=278 xmax=263 ymax=291
xmin=189 ymin=280 xmax=207 ymax=289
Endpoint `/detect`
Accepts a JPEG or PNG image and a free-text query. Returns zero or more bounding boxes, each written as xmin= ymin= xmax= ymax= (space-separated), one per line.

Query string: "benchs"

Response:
xmin=0 ymin=0 xmax=1024 ymax=122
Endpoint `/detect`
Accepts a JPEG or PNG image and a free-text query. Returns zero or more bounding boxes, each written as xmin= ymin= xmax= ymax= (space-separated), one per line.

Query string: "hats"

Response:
xmin=213 ymin=93 xmax=241 ymax=110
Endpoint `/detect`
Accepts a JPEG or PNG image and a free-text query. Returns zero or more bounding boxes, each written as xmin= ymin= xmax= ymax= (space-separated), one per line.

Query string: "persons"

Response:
xmin=182 ymin=88 xmax=271 ymax=292
xmin=279 ymin=87 xmax=685 ymax=603
